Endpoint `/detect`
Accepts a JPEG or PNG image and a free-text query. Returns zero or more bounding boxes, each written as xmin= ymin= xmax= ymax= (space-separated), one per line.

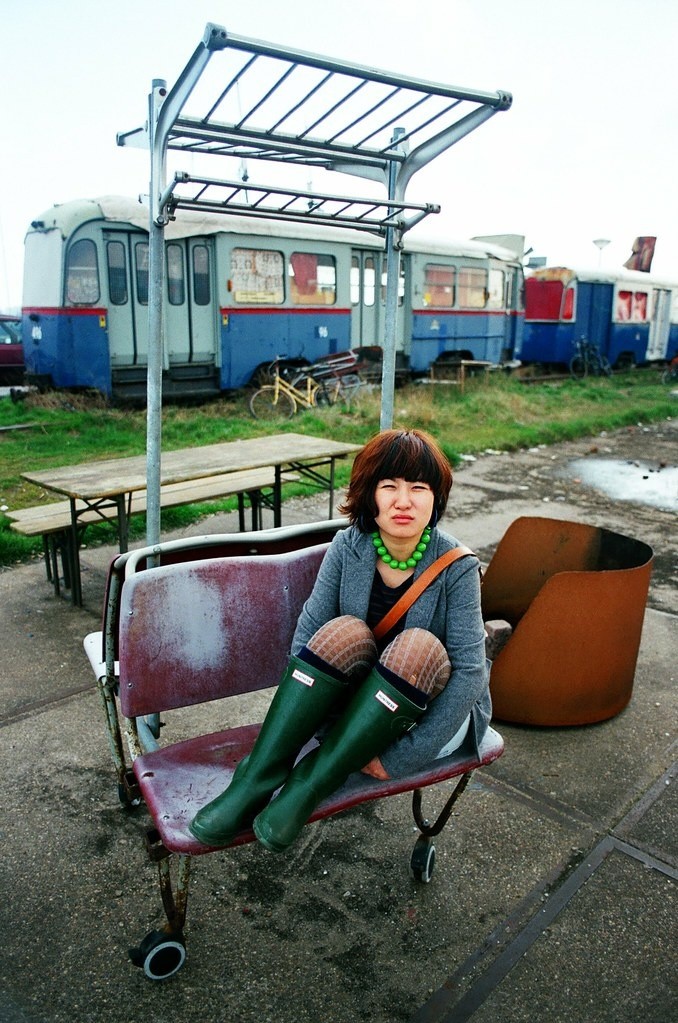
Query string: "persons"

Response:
xmin=187 ymin=427 xmax=495 ymax=856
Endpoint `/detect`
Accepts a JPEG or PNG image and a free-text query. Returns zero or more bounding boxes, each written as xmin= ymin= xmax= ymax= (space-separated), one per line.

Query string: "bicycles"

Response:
xmin=248 ymin=354 xmax=352 ymax=424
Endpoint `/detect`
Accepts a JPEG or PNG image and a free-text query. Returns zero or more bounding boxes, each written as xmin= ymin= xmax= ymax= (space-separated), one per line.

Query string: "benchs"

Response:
xmin=4 ymin=464 xmax=302 ymax=606
xmin=84 ymin=515 xmax=511 ymax=981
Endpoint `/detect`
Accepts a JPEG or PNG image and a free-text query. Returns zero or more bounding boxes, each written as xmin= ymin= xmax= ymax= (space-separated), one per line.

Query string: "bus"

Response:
xmin=516 ymin=258 xmax=678 ymax=374
xmin=21 ymin=190 xmax=528 ymax=408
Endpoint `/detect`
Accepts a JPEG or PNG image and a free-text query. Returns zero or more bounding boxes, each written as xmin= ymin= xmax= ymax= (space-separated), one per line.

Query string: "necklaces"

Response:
xmin=370 ymin=526 xmax=432 ymax=571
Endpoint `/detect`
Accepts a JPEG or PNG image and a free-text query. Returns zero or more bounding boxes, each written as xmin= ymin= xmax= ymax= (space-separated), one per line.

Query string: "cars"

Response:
xmin=0 ymin=314 xmax=22 ymax=386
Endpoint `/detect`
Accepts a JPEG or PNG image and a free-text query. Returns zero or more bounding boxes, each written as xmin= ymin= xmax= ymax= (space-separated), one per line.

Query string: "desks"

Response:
xmin=19 ymin=432 xmax=367 ymax=608
xmin=429 ymin=359 xmax=493 ymax=396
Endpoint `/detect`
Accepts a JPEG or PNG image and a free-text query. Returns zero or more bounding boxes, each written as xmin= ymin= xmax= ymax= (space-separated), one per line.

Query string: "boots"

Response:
xmin=252 ymin=665 xmax=427 ymax=855
xmin=187 ymin=654 xmax=350 ymax=846
